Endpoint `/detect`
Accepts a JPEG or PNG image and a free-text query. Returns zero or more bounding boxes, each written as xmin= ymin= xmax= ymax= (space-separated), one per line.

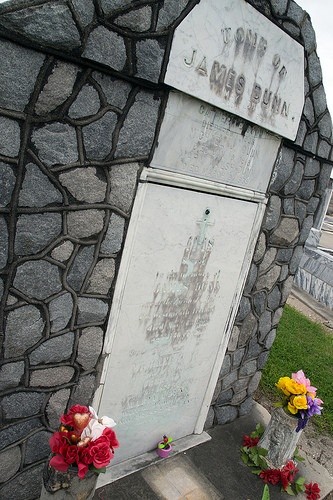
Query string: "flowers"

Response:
xmin=158 ymin=435 xmax=173 ymax=448
xmin=48 ymin=404 xmax=119 ymax=481
xmin=240 ymin=422 xmax=321 ymax=500
xmin=272 ymin=368 xmax=324 ymax=433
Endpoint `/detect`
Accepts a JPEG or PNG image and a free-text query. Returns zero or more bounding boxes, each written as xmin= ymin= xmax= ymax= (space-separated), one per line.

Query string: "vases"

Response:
xmin=256 ymin=406 xmax=303 ymax=471
xmin=156 ymin=441 xmax=172 ymax=459
xmin=39 ymin=450 xmax=100 ymax=500
